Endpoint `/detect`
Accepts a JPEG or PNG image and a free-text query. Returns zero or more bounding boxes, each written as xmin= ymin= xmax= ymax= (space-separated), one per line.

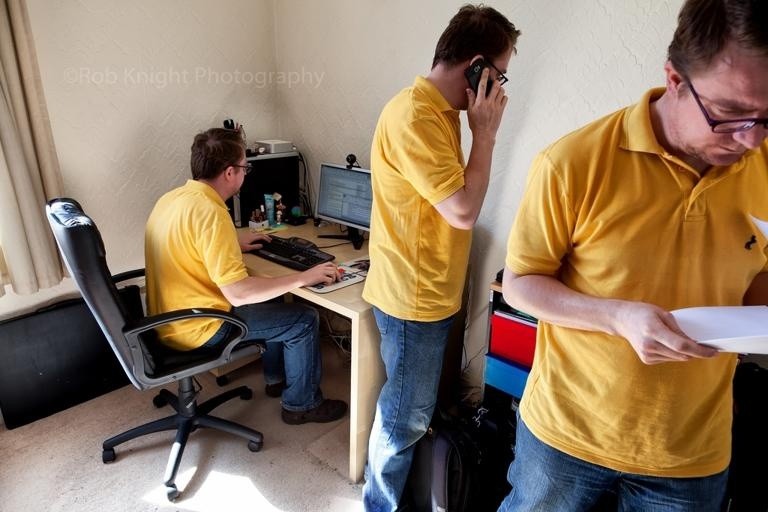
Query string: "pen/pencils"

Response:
xmin=261 ymin=204 xmax=266 ymax=221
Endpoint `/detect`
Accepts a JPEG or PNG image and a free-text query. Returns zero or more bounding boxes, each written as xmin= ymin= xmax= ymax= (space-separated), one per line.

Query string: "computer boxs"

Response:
xmin=225 ymin=151 xmax=300 ymax=228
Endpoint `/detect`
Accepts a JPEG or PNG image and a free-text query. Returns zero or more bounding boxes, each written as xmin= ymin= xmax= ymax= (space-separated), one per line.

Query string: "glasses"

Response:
xmin=484 ymin=57 xmax=508 ymax=86
xmin=225 ymin=163 xmax=253 ymax=175
xmin=675 ymin=58 xmax=768 ymax=134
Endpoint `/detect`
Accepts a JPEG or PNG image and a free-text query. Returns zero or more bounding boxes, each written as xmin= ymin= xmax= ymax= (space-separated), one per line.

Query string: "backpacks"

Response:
xmin=411 ymin=420 xmax=495 ymax=511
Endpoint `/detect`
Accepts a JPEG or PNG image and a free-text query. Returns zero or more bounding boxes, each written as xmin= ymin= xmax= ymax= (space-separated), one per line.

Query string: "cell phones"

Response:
xmin=464 ymin=59 xmax=494 ymax=98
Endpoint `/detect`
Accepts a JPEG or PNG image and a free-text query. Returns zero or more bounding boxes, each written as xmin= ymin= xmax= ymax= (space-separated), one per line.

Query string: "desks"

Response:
xmin=237 ymin=220 xmax=388 ymax=483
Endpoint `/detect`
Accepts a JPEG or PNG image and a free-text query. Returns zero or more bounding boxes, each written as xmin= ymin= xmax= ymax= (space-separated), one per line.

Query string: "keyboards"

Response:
xmin=245 ymin=234 xmax=335 ymax=271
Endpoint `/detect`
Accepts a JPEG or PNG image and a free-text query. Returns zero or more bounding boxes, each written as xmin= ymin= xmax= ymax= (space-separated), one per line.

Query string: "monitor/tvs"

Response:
xmin=314 ymin=161 xmax=373 ymax=250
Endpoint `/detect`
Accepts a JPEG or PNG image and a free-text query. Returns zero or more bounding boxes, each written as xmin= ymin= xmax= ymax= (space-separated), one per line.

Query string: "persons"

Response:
xmin=144 ymin=126 xmax=346 ymax=423
xmin=494 ymin=0 xmax=768 ymax=509
xmin=360 ymin=4 xmax=520 ymax=511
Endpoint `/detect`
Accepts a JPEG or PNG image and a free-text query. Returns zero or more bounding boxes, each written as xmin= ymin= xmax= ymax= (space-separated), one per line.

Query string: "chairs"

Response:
xmin=44 ymin=196 xmax=268 ymax=501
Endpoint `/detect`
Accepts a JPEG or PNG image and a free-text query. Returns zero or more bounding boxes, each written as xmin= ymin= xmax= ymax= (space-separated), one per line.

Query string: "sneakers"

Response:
xmin=265 ymin=380 xmax=288 ymax=398
xmin=280 ymin=398 xmax=348 ymax=425
